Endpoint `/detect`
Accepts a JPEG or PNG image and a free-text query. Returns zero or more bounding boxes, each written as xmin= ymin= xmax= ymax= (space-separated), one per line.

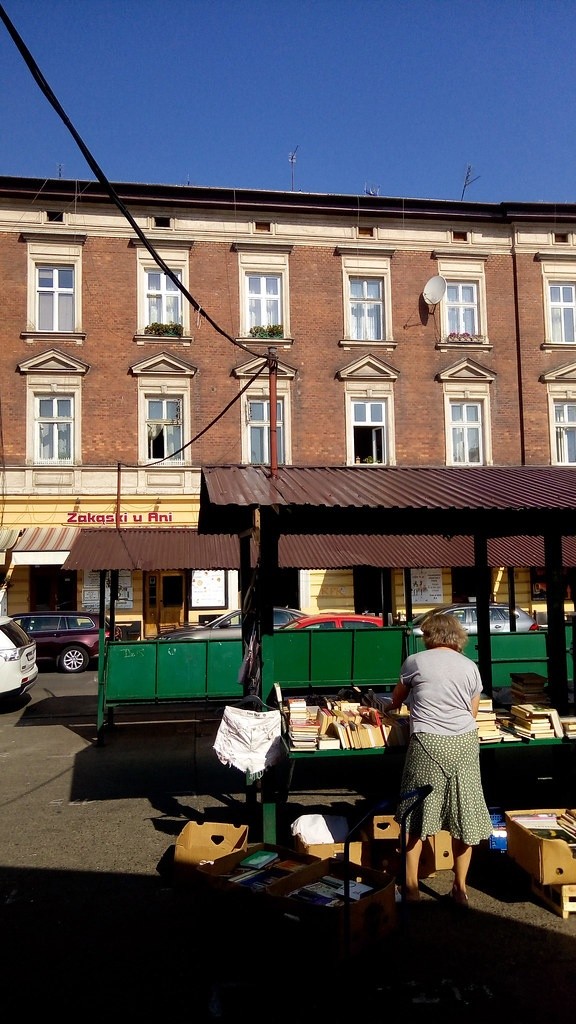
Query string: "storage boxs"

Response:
xmin=361 ymin=815 xmax=435 ymax=877
xmin=195 ymin=843 xmax=321 ymax=946
xmin=267 ymin=857 xmax=395 ymax=971
xmin=505 ymin=809 xmax=576 ymax=885
xmin=174 ymin=820 xmax=249 ymax=903
xmin=435 ymin=830 xmax=454 ymax=870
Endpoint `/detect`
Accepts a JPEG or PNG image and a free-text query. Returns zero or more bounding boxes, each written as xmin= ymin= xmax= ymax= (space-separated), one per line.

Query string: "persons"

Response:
xmin=386 ymin=615 xmax=496 ymax=912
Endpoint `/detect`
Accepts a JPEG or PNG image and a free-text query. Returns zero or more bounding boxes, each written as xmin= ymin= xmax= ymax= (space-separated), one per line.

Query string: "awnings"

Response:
xmin=0 ymin=527 xmax=85 ymax=567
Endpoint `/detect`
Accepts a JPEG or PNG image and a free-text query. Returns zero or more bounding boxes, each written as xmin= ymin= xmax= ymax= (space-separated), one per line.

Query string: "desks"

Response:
xmin=279 ymin=694 xmax=576 ymax=759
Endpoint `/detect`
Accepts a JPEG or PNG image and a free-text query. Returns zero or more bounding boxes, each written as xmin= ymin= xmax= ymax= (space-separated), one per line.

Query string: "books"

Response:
xmin=220 ymin=848 xmax=309 ymax=895
xmin=284 ymin=671 xmax=576 ymax=753
xmin=515 ymin=810 xmax=576 ymax=862
xmin=288 ymin=873 xmax=374 ymax=924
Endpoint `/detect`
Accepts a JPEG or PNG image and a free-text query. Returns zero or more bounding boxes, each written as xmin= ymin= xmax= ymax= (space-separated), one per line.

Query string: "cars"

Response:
xmin=5 ymin=611 xmax=125 ymax=673
xmin=152 ymin=608 xmax=311 ymax=647
xmin=408 ymin=601 xmax=537 ymax=633
xmin=0 ymin=614 xmax=39 ymax=700
xmin=279 ymin=612 xmax=394 ymax=634
